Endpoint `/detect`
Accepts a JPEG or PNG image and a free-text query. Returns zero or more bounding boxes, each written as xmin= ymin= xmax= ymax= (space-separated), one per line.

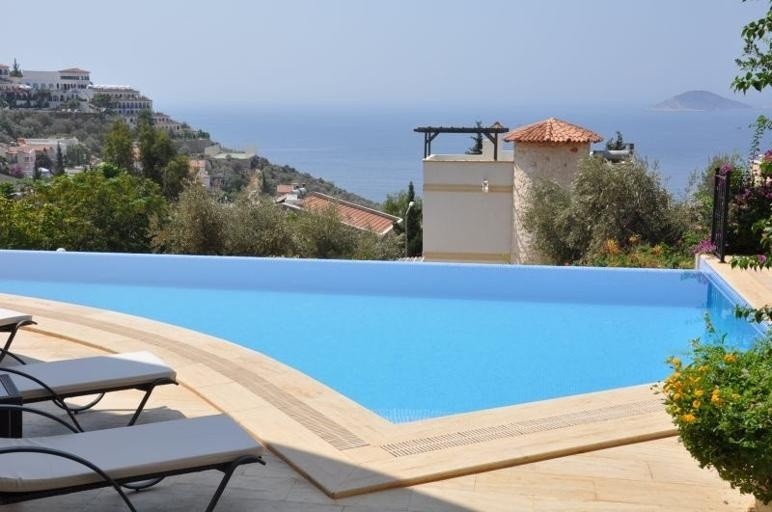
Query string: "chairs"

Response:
xmin=1 ymin=405 xmax=265 ymax=512
xmin=2 ymin=347 xmax=179 ymax=431
xmin=0 ymin=306 xmax=37 ymax=360
